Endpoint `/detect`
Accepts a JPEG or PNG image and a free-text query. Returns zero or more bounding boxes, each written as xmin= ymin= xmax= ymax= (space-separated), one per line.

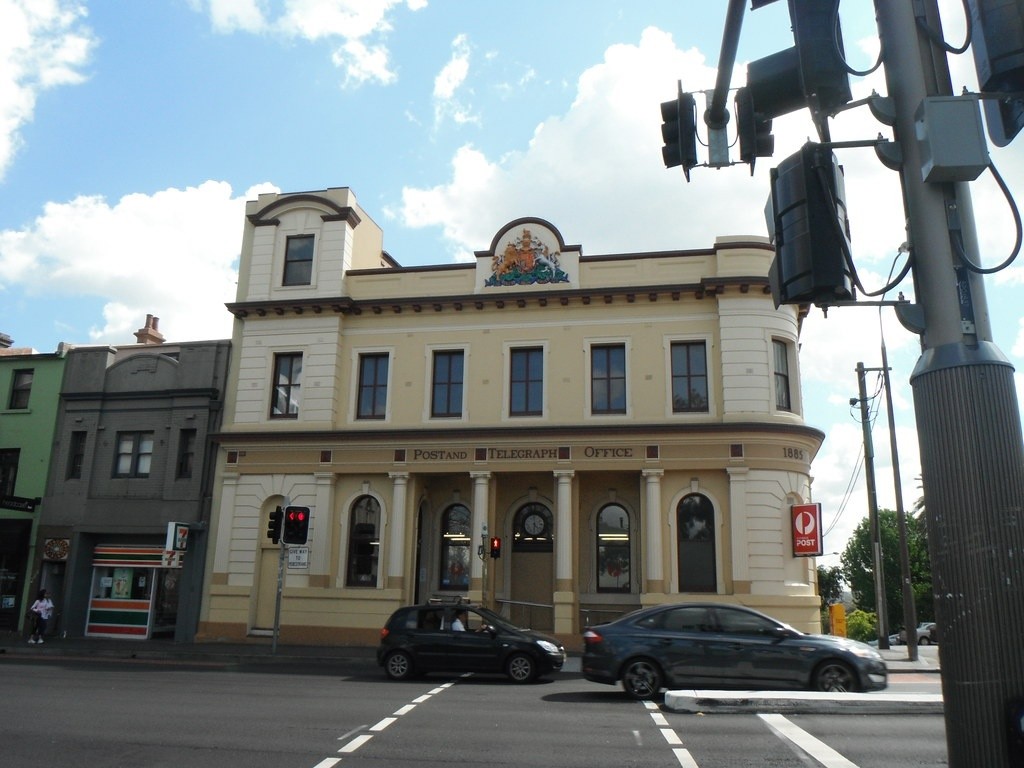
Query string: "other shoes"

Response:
xmin=27 ymin=639 xmax=36 ymax=644
xmin=37 ymin=639 xmax=44 ymax=643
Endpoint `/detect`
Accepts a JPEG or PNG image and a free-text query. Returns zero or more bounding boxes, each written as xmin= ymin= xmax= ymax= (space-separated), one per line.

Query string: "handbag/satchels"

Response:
xmin=25 ymin=607 xmax=39 ymax=625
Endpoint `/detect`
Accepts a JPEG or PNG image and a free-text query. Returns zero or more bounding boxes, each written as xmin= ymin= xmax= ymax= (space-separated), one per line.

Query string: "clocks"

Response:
xmin=523 ymin=512 xmax=547 ymax=537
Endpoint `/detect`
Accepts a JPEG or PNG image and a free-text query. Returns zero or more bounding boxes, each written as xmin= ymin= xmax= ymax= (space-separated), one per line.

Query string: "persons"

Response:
xmin=451 ymin=609 xmax=487 ymax=632
xmin=27 ymin=589 xmax=54 ymax=644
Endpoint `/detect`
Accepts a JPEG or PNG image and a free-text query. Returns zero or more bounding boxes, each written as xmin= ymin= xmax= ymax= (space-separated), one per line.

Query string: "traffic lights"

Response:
xmin=283 ymin=505 xmax=310 ymax=545
xmin=734 ymin=82 xmax=775 ymax=174
xmin=660 ymin=79 xmax=696 ymax=181
xmin=267 ymin=506 xmax=284 ymax=545
xmin=490 ymin=537 xmax=501 ymax=558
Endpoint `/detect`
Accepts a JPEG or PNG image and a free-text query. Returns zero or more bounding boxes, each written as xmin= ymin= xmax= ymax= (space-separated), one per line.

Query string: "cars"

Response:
xmin=374 ymin=594 xmax=567 ymax=683
xmin=868 ymin=621 xmax=938 ymax=646
xmin=581 ymin=601 xmax=889 ymax=699
